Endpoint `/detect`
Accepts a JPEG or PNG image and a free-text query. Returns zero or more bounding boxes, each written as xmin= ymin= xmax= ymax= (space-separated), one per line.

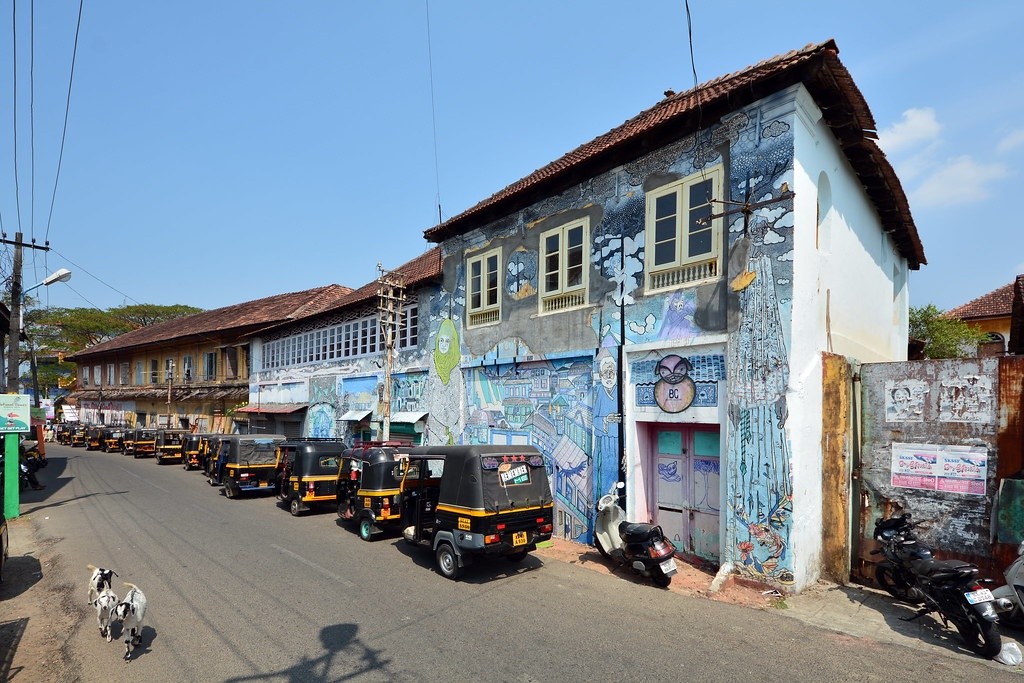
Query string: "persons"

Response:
xmin=19 ymin=456 xmax=47 ymax=490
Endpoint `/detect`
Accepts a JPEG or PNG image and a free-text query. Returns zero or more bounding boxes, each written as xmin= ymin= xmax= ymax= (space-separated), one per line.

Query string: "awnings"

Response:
xmin=371 ymin=412 xmax=430 ymax=424
xmin=335 ymin=410 xmax=374 ymax=422
xmin=234 ymin=403 xmax=309 ymax=414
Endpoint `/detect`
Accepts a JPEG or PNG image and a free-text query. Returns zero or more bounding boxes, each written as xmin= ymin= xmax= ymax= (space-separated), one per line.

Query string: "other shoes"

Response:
xmin=34 ymin=484 xmax=46 ymax=490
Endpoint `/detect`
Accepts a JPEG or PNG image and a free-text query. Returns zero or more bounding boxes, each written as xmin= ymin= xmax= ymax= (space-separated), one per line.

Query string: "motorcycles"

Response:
xmin=333 ymin=441 xmax=431 ymax=542
xmin=397 ymin=445 xmax=552 ymax=579
xmin=869 ymin=511 xmax=1013 ymax=659
xmin=273 ymin=437 xmax=348 ymax=516
xmin=19 ymin=456 xmax=34 ymax=494
xmin=57 ymin=424 xmax=285 ymax=498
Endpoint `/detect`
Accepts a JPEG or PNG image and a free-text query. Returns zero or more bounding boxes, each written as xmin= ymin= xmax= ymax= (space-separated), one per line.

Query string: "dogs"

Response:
xmin=109 ymin=583 xmax=147 ymax=659
xmin=93 ymin=587 xmax=118 ymax=637
xmin=86 ymin=565 xmax=118 ymax=606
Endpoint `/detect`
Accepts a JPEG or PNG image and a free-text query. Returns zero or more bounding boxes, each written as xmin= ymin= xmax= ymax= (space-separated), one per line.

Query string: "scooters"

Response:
xmin=587 ymin=481 xmax=678 ymax=587
xmin=991 ymin=540 xmax=1024 ymax=629
